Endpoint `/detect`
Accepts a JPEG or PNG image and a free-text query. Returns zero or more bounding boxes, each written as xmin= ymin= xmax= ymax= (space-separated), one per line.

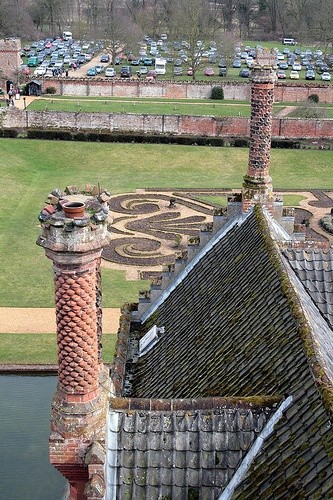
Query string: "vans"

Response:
xmin=173 ymin=67 xmax=183 ymax=77
xmin=120 ymin=66 xmax=132 ymax=77
xmin=94 ymin=64 xmax=104 ymax=74
xmin=282 ymin=38 xmax=298 ymax=46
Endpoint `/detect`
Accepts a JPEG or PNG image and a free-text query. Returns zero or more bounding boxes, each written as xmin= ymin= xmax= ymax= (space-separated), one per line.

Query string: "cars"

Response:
xmin=19 ymin=31 xmax=333 ymax=81
xmin=289 ymin=71 xmax=299 ymax=80
xmin=87 ymin=67 xmax=97 ymax=77
xmin=232 ymin=59 xmax=241 ymax=68
xmin=305 ymin=70 xmax=316 ymax=80
xmin=320 ymin=72 xmax=332 ymax=81
xmin=291 ymin=61 xmax=302 ymax=71
xmin=276 ymin=70 xmax=287 ymax=79
xmin=219 ymin=68 xmax=227 ymax=76
xmin=203 ymin=68 xmax=214 ymax=76
xmin=279 ymin=62 xmax=289 ymax=70
xmin=104 ymin=67 xmax=116 ymax=77
xmin=239 ymin=69 xmax=250 ymax=78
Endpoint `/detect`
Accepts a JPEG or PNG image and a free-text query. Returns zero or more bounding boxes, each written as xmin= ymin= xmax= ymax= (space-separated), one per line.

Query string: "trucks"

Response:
xmin=154 ymin=59 xmax=167 ymax=76
